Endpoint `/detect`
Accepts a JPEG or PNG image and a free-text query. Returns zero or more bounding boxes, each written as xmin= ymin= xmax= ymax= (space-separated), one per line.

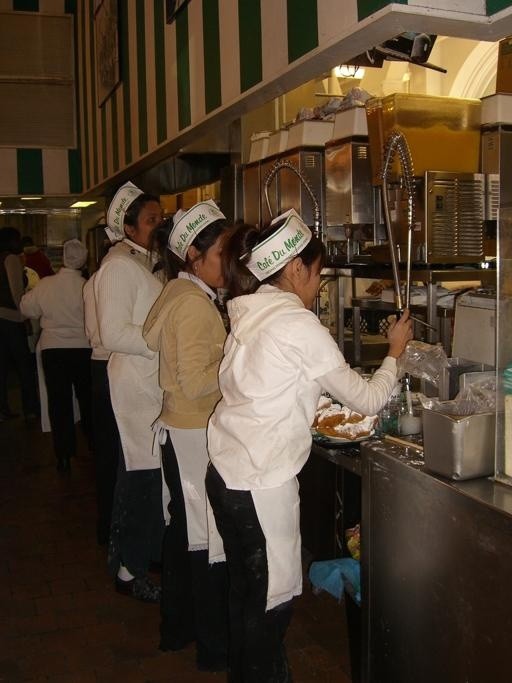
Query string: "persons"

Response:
xmin=208 ymin=215 xmax=408 ymax=680
xmin=0 ymin=228 xmax=99 ymax=476
xmin=144 ymin=200 xmax=244 ymax=671
xmin=97 ymin=182 xmax=178 ymax=600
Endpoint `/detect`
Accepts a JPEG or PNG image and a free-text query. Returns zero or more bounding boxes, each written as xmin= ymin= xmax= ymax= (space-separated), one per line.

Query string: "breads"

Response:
xmin=312 ymin=395 xmax=378 ymax=440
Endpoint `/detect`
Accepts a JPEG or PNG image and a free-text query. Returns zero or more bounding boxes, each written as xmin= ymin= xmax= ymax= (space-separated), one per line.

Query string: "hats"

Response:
xmin=63 ymin=239 xmax=88 ymax=268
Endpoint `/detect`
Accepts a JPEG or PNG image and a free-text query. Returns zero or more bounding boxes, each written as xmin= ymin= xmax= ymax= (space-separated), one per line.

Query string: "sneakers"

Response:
xmin=116 ymin=575 xmax=162 ymax=601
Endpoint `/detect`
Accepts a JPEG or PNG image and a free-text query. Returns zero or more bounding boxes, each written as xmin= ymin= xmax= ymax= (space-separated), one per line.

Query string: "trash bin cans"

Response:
xmin=343 ymin=583 xmax=363 ymax=683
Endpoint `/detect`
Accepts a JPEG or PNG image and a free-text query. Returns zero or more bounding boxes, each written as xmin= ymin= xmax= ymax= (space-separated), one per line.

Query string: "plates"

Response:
xmin=323 ymin=429 xmax=375 ymax=444
